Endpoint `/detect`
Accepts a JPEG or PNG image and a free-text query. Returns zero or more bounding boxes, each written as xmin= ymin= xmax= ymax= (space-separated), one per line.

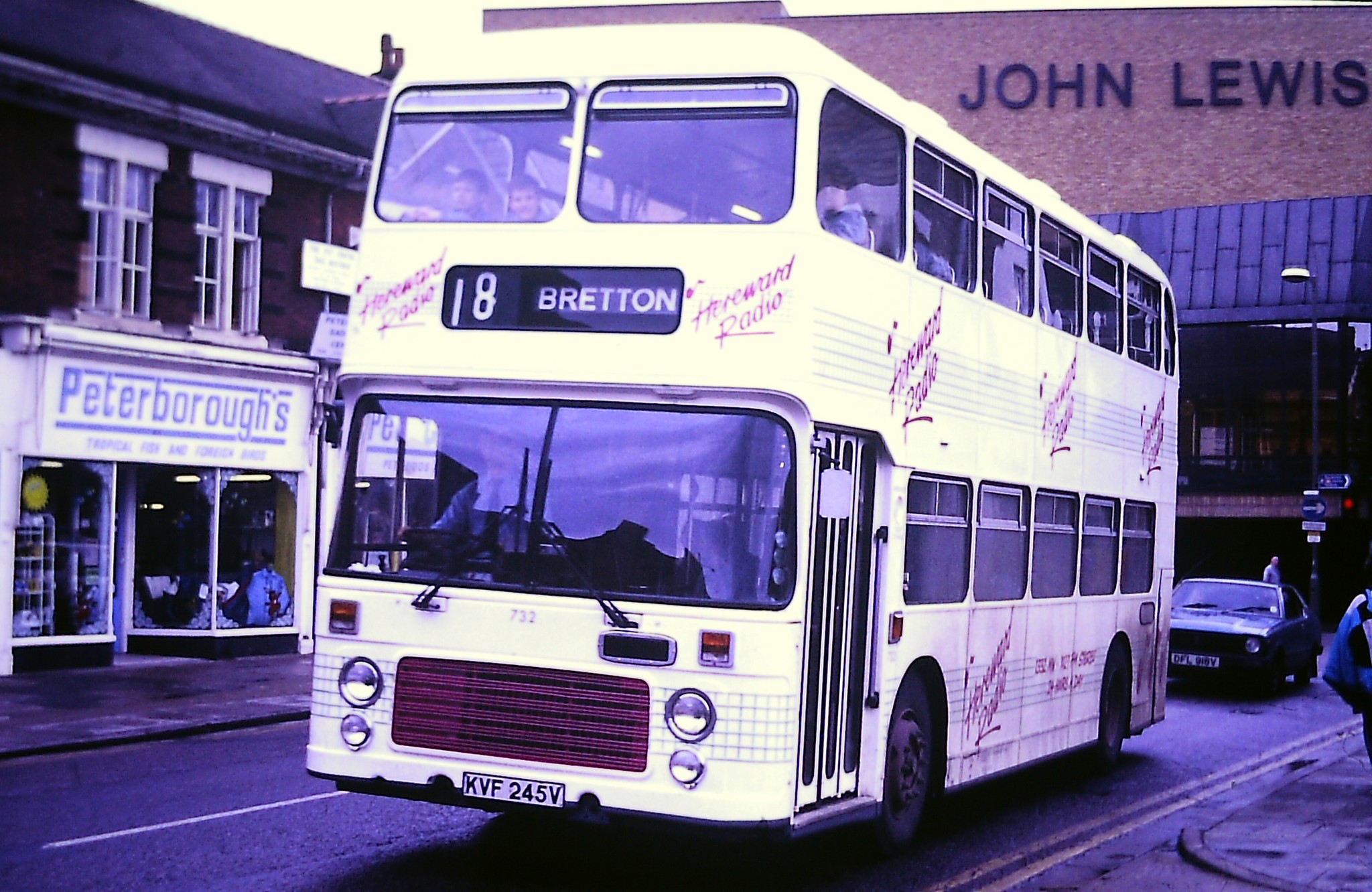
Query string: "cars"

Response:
xmin=1171 ymin=577 xmax=1324 ymax=696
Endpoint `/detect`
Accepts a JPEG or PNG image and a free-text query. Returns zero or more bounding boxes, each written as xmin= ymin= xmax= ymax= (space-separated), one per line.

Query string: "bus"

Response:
xmin=303 ymin=22 xmax=1180 ymax=859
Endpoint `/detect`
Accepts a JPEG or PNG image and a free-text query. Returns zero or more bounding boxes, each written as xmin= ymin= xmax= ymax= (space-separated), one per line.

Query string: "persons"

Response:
xmin=509 ymin=175 xmax=550 ymax=222
xmin=1263 ymin=556 xmax=1280 ymax=583
xmin=1323 ymin=589 xmax=1372 ymax=764
xmin=397 ymin=418 xmax=536 ymax=552
xmin=820 ymin=162 xmax=869 ymax=246
xmin=452 ymin=169 xmax=488 ymax=219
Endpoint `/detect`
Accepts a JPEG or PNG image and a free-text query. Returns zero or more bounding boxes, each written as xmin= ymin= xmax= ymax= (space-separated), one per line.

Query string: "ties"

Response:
xmin=485 ymin=477 xmax=502 ymax=542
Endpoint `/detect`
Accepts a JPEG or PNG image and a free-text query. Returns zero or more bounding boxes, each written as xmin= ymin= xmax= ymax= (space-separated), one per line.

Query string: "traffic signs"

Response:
xmin=1318 ymin=473 xmax=1352 ymax=489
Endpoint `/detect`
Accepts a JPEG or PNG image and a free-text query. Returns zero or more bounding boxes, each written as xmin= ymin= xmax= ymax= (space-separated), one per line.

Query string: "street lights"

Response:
xmin=1279 ymin=266 xmax=1321 ymax=618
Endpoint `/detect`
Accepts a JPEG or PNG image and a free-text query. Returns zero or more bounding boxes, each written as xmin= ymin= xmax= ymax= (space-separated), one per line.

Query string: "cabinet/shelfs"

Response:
xmin=14 ymin=513 xmax=54 ymax=638
